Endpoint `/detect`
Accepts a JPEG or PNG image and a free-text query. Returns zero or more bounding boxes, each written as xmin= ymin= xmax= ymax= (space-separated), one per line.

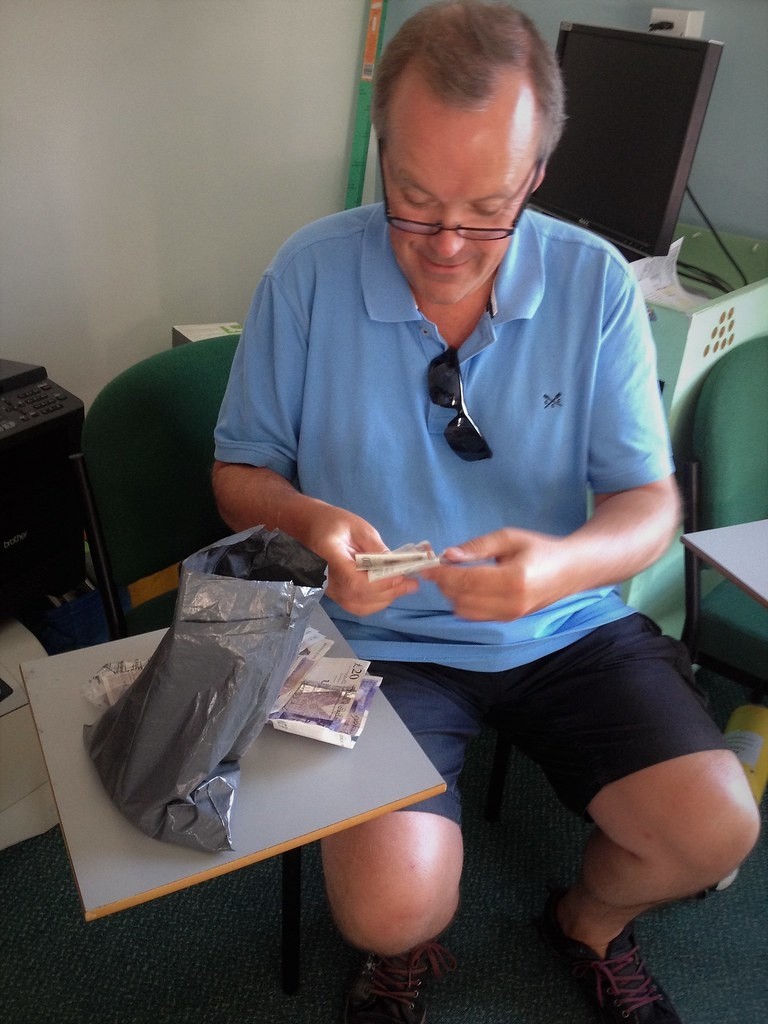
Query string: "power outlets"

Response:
xmin=646 ymin=7 xmax=707 ymax=39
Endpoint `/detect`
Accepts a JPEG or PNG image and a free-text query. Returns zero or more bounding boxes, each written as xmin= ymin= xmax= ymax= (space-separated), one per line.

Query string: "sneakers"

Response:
xmin=343 ymin=934 xmax=457 ymax=1024
xmin=539 ymin=878 xmax=681 ymax=1023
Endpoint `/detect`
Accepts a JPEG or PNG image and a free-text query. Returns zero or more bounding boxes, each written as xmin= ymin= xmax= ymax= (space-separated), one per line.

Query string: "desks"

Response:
xmin=18 ymin=597 xmax=447 ymax=924
xmin=677 ymin=519 xmax=767 ymax=611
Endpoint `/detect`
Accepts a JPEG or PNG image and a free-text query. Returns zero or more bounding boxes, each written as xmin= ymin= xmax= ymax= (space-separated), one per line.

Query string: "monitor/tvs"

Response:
xmin=520 ymin=19 xmax=725 ymax=266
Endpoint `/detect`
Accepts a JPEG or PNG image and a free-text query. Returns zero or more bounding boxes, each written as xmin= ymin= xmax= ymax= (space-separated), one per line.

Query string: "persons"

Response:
xmin=216 ymin=0 xmax=760 ymax=1023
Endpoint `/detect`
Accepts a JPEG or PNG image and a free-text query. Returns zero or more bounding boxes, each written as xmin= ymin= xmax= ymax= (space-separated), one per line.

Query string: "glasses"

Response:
xmin=378 ymin=137 xmax=544 ymax=240
xmin=428 ymin=345 xmax=493 ymax=461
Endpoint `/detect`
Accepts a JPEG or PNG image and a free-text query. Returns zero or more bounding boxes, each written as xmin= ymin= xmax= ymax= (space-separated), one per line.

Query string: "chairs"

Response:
xmin=82 ymin=339 xmax=515 ymax=993
xmin=658 ymin=339 xmax=768 ymax=718
